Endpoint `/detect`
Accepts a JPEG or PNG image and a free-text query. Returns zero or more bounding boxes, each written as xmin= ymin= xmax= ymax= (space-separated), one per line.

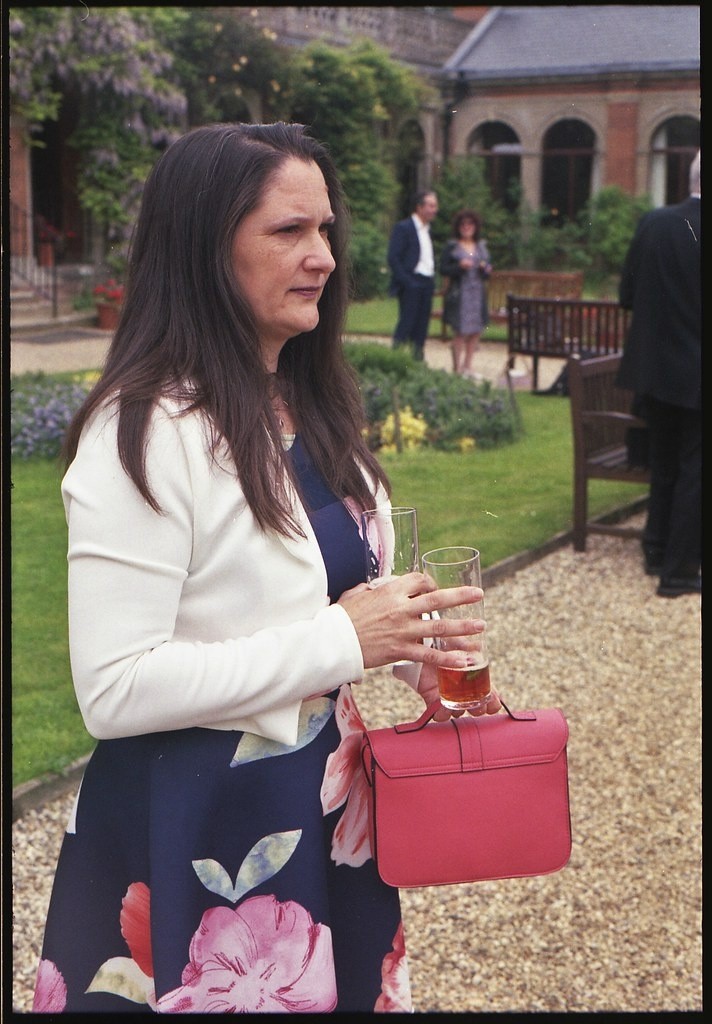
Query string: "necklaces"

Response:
xmin=278 ymin=394 xmax=285 ymax=427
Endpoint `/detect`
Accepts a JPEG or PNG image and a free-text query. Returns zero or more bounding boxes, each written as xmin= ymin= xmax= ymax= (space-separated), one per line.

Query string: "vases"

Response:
xmin=36 ymin=243 xmax=55 ymax=267
xmin=95 ymin=303 xmax=124 ymax=330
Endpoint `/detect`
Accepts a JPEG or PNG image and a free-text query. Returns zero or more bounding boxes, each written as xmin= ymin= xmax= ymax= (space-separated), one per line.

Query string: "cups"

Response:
xmin=363 ymin=507 xmax=425 ymax=665
xmin=422 ymin=545 xmax=490 ymax=710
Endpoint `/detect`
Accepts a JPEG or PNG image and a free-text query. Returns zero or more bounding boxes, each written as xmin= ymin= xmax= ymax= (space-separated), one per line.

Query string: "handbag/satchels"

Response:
xmin=362 ymin=698 xmax=572 ymax=888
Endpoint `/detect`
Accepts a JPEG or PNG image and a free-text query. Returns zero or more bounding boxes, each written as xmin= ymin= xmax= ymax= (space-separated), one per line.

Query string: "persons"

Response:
xmin=438 ymin=209 xmax=494 ymax=374
xmin=32 ymin=121 xmax=500 ymax=1013
xmin=614 ymin=149 xmax=704 ymax=598
xmin=388 ymin=188 xmax=439 ymax=352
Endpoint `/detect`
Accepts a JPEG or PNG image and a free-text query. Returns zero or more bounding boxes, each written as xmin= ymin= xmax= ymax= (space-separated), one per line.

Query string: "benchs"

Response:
xmin=430 ymin=268 xmax=583 ymax=343
xmin=566 ymin=352 xmax=651 ymax=554
xmin=504 ymin=293 xmax=634 ymax=394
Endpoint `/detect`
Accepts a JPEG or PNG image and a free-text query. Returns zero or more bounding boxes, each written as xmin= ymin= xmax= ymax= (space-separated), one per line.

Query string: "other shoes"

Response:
xmin=657 ymin=579 xmax=701 ymax=597
xmin=463 ymin=366 xmax=478 ymax=380
xmin=643 ymin=559 xmax=665 ymax=576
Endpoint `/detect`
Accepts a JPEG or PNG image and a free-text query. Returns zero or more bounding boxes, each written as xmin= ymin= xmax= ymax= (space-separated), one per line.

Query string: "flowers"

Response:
xmin=33 ymin=213 xmax=64 ymax=251
xmin=93 ymin=278 xmax=126 ymax=305
